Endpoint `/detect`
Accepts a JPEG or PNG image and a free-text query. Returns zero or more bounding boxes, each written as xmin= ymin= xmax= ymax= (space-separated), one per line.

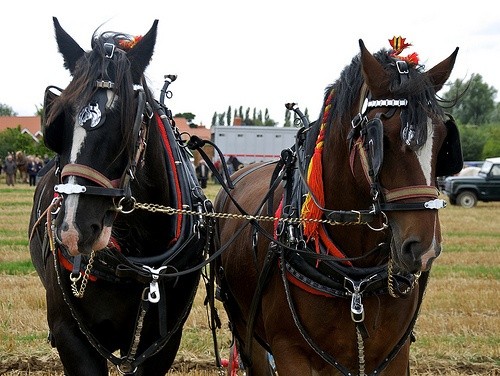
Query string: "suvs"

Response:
xmin=445 ymin=157 xmax=500 ymax=206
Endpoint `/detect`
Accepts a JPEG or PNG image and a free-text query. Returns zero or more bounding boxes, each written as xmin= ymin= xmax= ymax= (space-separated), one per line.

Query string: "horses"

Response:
xmin=28 ymin=16 xmax=207 ymax=376
xmin=214 ymin=39 xmax=473 ymax=376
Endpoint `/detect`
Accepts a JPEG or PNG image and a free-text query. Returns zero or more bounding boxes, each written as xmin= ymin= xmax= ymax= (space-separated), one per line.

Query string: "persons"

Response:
xmin=4 ymin=155 xmax=17 ymax=186
xmin=27 ymin=157 xmax=38 ymax=186
xmin=0 ymin=156 xmax=4 ymax=175
xmin=35 ymin=158 xmax=43 ymax=180
xmin=194 ymin=156 xmax=225 ymax=190
